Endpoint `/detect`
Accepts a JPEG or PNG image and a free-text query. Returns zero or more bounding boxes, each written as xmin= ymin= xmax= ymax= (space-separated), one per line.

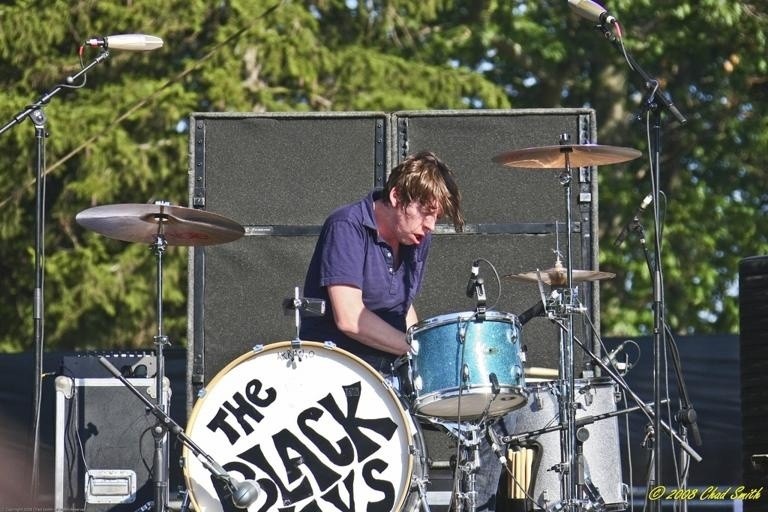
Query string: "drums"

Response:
xmin=501 ymin=379 xmax=628 ymax=511
xmin=406 ymin=312 xmax=529 ymax=420
xmin=181 ymin=341 xmax=428 ymax=512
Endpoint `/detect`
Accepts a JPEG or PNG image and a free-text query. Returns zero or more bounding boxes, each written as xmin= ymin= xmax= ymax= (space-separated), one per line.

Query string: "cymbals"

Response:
xmin=502 ymin=268 xmax=616 ymax=285
xmin=492 ymin=144 xmax=642 ymax=169
xmin=76 ymin=203 xmax=245 ymax=245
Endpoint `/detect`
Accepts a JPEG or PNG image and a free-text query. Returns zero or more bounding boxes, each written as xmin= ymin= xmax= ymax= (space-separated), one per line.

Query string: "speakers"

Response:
xmin=739 ymin=256 xmax=768 ymax=512
xmin=392 ymin=107 xmax=602 ymax=480
xmin=185 ymin=112 xmax=391 ymax=420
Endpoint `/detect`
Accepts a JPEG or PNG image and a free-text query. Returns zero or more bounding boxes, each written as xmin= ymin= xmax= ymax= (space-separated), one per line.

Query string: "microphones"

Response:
xmin=612 ymin=193 xmax=653 ymax=248
xmin=85 ymin=32 xmax=163 ymax=52
xmin=600 ymin=344 xmax=622 ymax=369
xmin=564 ymin=0 xmax=616 ymax=25
xmin=466 ymin=261 xmax=479 ymax=298
xmin=229 ymin=477 xmax=260 ymax=508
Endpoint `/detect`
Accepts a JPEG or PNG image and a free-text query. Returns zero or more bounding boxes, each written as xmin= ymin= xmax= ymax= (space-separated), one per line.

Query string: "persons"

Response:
xmin=299 ymin=151 xmax=508 ymax=512
